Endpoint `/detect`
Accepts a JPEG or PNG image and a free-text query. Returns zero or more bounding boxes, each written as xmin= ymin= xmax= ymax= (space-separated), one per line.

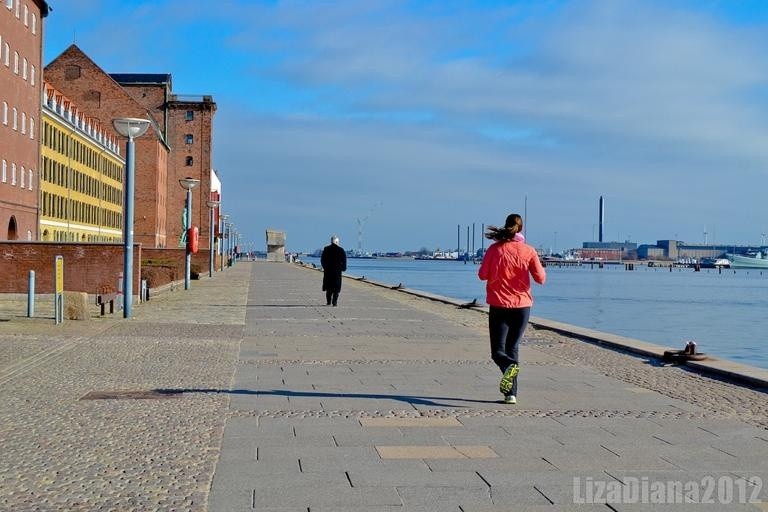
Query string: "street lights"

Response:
xmin=206 ymin=201 xmax=219 ymax=277
xmin=179 ymin=177 xmax=200 ymax=290
xmin=225 ymin=223 xmax=239 ymax=267
xmin=111 ymin=117 xmax=151 ymax=318
xmin=219 ymin=215 xmax=229 ymax=271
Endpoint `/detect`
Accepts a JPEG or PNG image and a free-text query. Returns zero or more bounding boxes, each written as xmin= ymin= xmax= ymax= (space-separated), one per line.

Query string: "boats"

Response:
xmin=725 ymin=231 xmax=768 ymax=269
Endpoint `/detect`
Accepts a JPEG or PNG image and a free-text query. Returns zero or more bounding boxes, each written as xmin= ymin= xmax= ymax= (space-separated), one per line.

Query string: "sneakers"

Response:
xmin=499 ymin=362 xmax=520 ymax=394
xmin=503 ymin=394 xmax=517 ymax=404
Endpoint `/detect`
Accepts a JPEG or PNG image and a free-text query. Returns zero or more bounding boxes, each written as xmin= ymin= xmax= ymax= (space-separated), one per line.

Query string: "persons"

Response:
xmin=320 ymin=235 xmax=348 ymax=307
xmin=477 ymin=214 xmax=546 ymax=404
